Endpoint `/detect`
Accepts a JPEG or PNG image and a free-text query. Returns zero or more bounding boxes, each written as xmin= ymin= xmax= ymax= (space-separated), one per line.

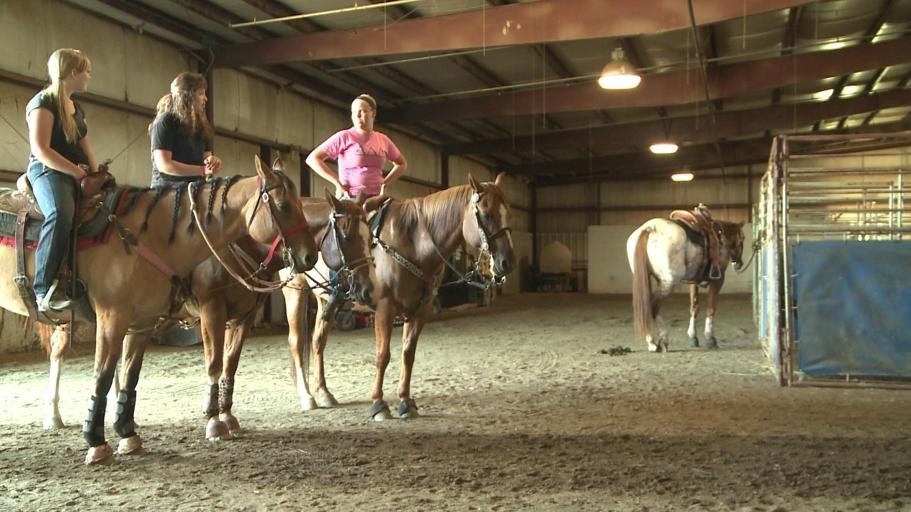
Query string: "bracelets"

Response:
xmin=201 ymin=166 xmax=204 ymax=176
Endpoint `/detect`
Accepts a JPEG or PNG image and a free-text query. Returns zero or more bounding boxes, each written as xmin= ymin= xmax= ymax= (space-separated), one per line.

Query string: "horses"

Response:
xmin=278 ymin=171 xmax=518 ymax=422
xmin=18 ymin=185 xmax=390 ymax=439
xmin=625 ymin=217 xmax=746 ymax=353
xmin=0 ymin=152 xmax=320 ymax=464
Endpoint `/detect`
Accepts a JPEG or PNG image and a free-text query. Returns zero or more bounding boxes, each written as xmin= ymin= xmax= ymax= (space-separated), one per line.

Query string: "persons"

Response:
xmin=151 ymin=72 xmax=222 ymax=190
xmin=304 ymin=93 xmax=408 ymax=202
xmin=26 ymin=48 xmax=100 ymax=312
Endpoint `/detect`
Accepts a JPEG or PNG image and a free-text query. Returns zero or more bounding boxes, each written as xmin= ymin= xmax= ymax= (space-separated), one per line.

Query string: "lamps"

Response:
xmin=649 ymin=111 xmax=679 ymax=156
xmin=596 ymin=43 xmax=644 ymax=93
xmin=670 ymin=160 xmax=695 ymax=184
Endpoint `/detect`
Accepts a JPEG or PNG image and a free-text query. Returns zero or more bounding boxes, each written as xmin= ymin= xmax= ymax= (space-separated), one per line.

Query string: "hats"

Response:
xmin=355 ymin=94 xmax=377 ymax=109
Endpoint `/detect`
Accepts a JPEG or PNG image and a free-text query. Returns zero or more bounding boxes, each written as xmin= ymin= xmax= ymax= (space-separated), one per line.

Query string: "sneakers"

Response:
xmin=36 ymin=293 xmax=71 ymax=312
